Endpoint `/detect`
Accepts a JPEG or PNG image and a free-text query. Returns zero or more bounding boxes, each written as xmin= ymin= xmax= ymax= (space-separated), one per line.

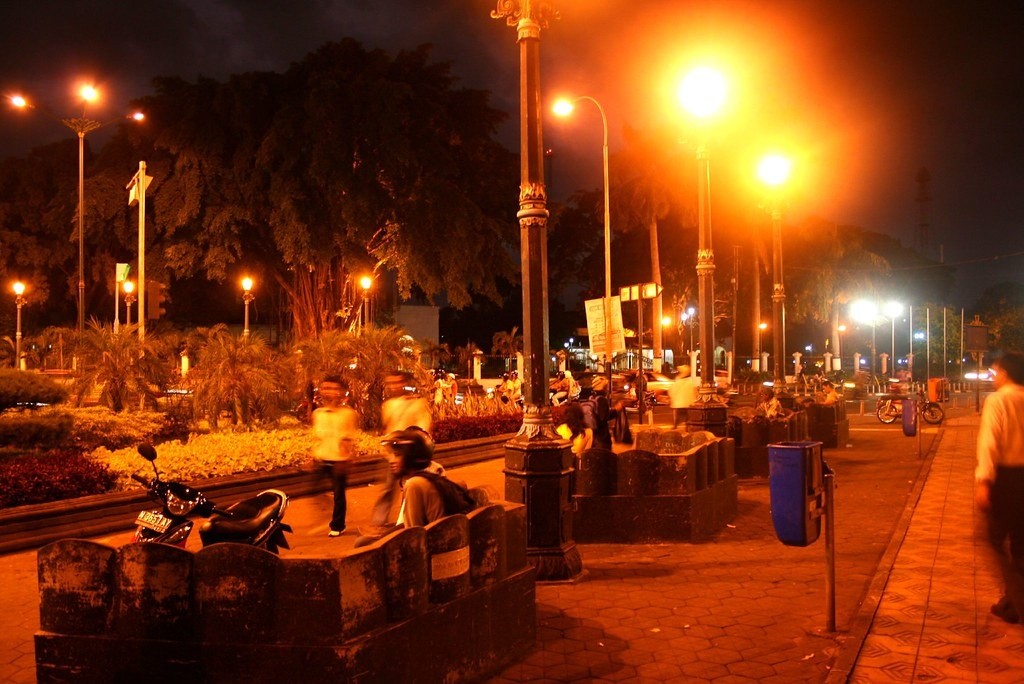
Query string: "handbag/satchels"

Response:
xmin=614 ymin=406 xmax=633 ymax=444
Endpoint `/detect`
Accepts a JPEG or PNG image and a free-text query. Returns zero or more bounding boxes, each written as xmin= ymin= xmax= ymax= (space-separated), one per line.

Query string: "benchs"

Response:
xmin=729 ymin=405 xmax=808 ymax=479
xmin=572 ymin=431 xmax=739 ymax=546
xmin=807 ymin=399 xmax=851 ymax=449
xmin=33 ymin=497 xmax=537 ymax=684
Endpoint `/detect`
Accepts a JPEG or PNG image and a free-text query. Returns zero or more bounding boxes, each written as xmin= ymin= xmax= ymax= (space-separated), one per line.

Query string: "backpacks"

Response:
xmin=580 ymin=395 xmax=605 ymax=432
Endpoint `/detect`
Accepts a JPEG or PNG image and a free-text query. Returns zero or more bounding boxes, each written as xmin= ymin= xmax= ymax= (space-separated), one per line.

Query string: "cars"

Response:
xmin=627 ymin=369 xmax=676 ymax=405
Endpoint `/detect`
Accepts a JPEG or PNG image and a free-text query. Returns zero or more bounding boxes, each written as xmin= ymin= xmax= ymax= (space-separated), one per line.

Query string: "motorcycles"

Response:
xmin=876 ymin=388 xmax=945 ymax=425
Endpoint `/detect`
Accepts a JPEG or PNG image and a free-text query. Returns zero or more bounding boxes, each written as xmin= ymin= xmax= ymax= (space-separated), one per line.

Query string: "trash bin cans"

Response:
xmin=902 ymin=400 xmax=917 ymax=436
xmin=765 ymin=439 xmax=824 ymax=546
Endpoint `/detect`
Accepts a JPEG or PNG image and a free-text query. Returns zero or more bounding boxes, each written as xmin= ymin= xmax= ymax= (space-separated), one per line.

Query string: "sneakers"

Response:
xmin=328 ymin=528 xmax=346 ymax=538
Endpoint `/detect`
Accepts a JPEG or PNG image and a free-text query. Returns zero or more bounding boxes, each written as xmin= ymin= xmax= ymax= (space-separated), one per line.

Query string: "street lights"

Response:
xmin=242 ymin=279 xmax=253 ymax=337
xmin=124 ymin=282 xmax=135 ymax=325
xmin=758 ymin=323 xmax=768 ymax=374
xmin=360 ymin=277 xmax=372 ymax=326
xmin=552 ymin=97 xmax=612 ymax=390
xmin=678 ymin=64 xmax=731 ymax=432
xmin=687 ymin=307 xmax=696 ymax=352
xmin=12 ymin=87 xmax=144 ymax=397
xmin=13 ymin=282 xmax=26 ymax=369
xmin=752 ymin=150 xmax=798 ymax=412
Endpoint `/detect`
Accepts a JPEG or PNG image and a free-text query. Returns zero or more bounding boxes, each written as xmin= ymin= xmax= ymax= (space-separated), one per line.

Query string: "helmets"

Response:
xmin=760 ymin=388 xmax=772 ymax=397
xmin=556 ymin=371 xmax=565 ymax=379
xmin=636 ymin=367 xmax=644 ymax=375
xmin=381 ymin=430 xmax=436 ymax=469
xmin=502 ymin=372 xmax=510 ymax=379
xmin=448 ymin=373 xmax=455 ymax=380
xmin=591 ymin=376 xmax=610 ymax=393
xmin=677 ymin=365 xmax=690 ymax=376
xmin=564 ymin=370 xmax=572 ymax=378
xmin=436 ymin=368 xmax=446 ymax=378
xmin=510 ymin=370 xmax=518 ymax=379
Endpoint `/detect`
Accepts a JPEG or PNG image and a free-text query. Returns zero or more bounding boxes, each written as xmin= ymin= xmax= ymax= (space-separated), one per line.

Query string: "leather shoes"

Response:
xmin=991 ymin=597 xmax=1019 ymax=624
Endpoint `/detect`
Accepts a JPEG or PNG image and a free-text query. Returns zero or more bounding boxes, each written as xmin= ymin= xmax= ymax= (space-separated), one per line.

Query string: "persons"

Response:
xmin=430 ymin=368 xmax=458 ymax=405
xmin=669 ymin=364 xmax=695 ymax=428
xmin=549 ymin=370 xmax=647 ymax=471
xmin=817 ymin=381 xmax=840 ymax=406
xmin=355 ymin=370 xmax=476 ymax=603
xmin=494 ymin=370 xmax=521 ymax=410
xmin=754 ymin=387 xmax=784 ymax=418
xmin=807 ymin=370 xmax=829 ymax=385
xmin=974 ymin=349 xmax=1024 ymax=623
xmin=310 ymin=376 xmax=361 ymax=536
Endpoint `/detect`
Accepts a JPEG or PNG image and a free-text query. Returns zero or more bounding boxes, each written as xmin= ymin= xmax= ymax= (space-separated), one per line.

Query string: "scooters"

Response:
xmin=628 ymin=387 xmax=657 ymax=415
xmin=124 ymin=442 xmax=293 ymax=555
